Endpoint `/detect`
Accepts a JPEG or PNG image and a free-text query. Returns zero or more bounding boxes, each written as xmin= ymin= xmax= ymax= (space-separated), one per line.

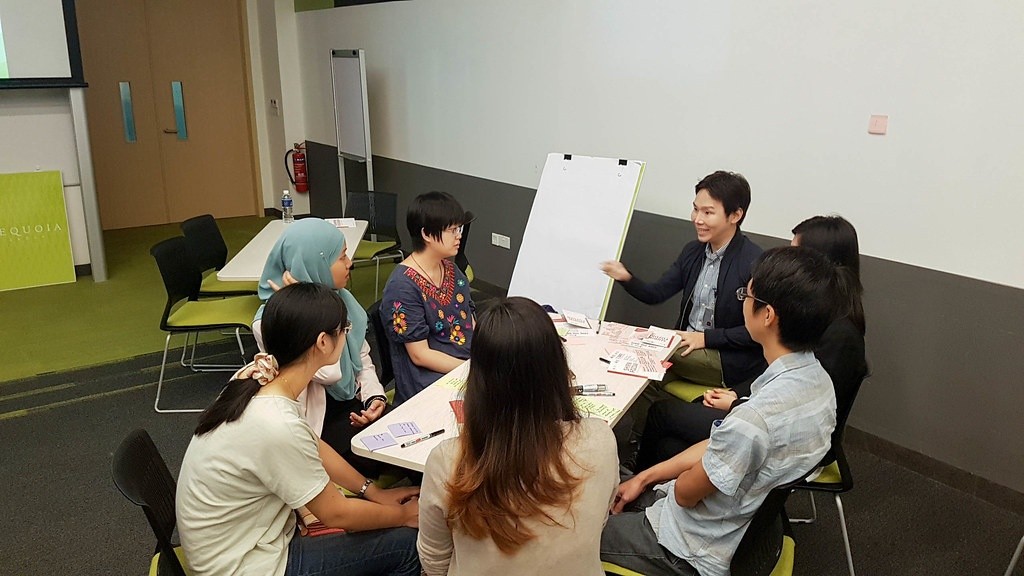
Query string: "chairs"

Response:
xmin=180 ymin=214 xmax=259 ymax=367
xmin=790 ymin=356 xmax=873 ymax=576
xmin=149 ymin=235 xmax=265 ymax=414
xmin=649 ymin=377 xmax=734 ymax=405
xmin=342 ymin=190 xmax=404 ymax=304
xmin=601 ymin=433 xmax=839 ymax=576
xmin=111 ymin=429 xmax=186 ymax=576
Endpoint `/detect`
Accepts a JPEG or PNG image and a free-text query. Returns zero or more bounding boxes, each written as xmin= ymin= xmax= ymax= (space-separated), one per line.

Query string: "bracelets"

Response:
xmin=357 ymin=479 xmax=373 ymax=498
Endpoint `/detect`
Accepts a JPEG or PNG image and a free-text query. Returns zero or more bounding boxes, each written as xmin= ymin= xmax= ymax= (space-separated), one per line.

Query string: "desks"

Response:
xmin=350 ymin=312 xmax=682 ymax=473
xmin=216 ymin=219 xmax=368 ymax=281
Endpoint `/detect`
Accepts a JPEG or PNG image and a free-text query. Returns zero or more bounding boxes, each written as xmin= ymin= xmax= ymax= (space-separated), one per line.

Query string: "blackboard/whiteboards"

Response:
xmin=324 ymin=48 xmax=373 ymax=163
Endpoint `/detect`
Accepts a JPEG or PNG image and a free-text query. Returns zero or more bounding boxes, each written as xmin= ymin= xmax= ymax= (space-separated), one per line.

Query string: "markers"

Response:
xmin=574 ymin=384 xmax=606 ymax=392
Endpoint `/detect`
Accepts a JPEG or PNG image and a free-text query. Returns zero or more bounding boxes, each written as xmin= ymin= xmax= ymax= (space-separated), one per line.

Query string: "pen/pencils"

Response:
xmin=401 ymin=429 xmax=445 ymax=449
xmin=575 ymin=392 xmax=615 ymax=396
xmin=596 ymin=320 xmax=602 ymax=334
xmin=599 ymin=358 xmax=611 ymax=364
xmin=560 ymin=336 xmax=567 ymax=342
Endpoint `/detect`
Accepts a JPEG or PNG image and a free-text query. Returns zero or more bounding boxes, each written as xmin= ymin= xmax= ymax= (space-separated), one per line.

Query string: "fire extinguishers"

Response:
xmin=284 ymin=141 xmax=310 ymax=194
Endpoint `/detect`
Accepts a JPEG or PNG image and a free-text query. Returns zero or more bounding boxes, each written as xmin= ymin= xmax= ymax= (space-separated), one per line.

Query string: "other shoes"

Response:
xmin=618 ymin=445 xmax=643 ymax=476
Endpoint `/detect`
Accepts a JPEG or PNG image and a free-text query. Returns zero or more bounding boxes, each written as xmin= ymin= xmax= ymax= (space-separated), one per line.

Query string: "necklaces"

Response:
xmin=411 ymin=253 xmax=442 ymax=292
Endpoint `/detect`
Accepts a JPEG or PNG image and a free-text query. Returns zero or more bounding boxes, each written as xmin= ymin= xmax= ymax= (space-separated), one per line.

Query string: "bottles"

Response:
xmin=281 ymin=189 xmax=294 ymax=223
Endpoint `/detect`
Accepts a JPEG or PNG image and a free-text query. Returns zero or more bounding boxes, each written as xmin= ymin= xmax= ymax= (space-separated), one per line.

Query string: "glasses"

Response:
xmin=735 ymin=286 xmax=780 ymax=318
xmin=334 ymin=319 xmax=353 ymax=334
xmin=443 ymin=224 xmax=465 ymax=237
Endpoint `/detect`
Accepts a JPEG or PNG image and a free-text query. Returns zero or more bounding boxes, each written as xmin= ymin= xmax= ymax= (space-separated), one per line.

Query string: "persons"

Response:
xmin=379 ymin=191 xmax=478 ymax=407
xmin=633 ymin=216 xmax=866 ymax=477
xmin=598 ymin=171 xmax=769 ymax=476
xmin=599 ymin=245 xmax=844 ymax=576
xmin=415 ymin=297 xmax=621 ymax=576
xmin=175 ymin=282 xmax=420 ymax=576
xmin=251 ymin=218 xmax=423 ymax=484
